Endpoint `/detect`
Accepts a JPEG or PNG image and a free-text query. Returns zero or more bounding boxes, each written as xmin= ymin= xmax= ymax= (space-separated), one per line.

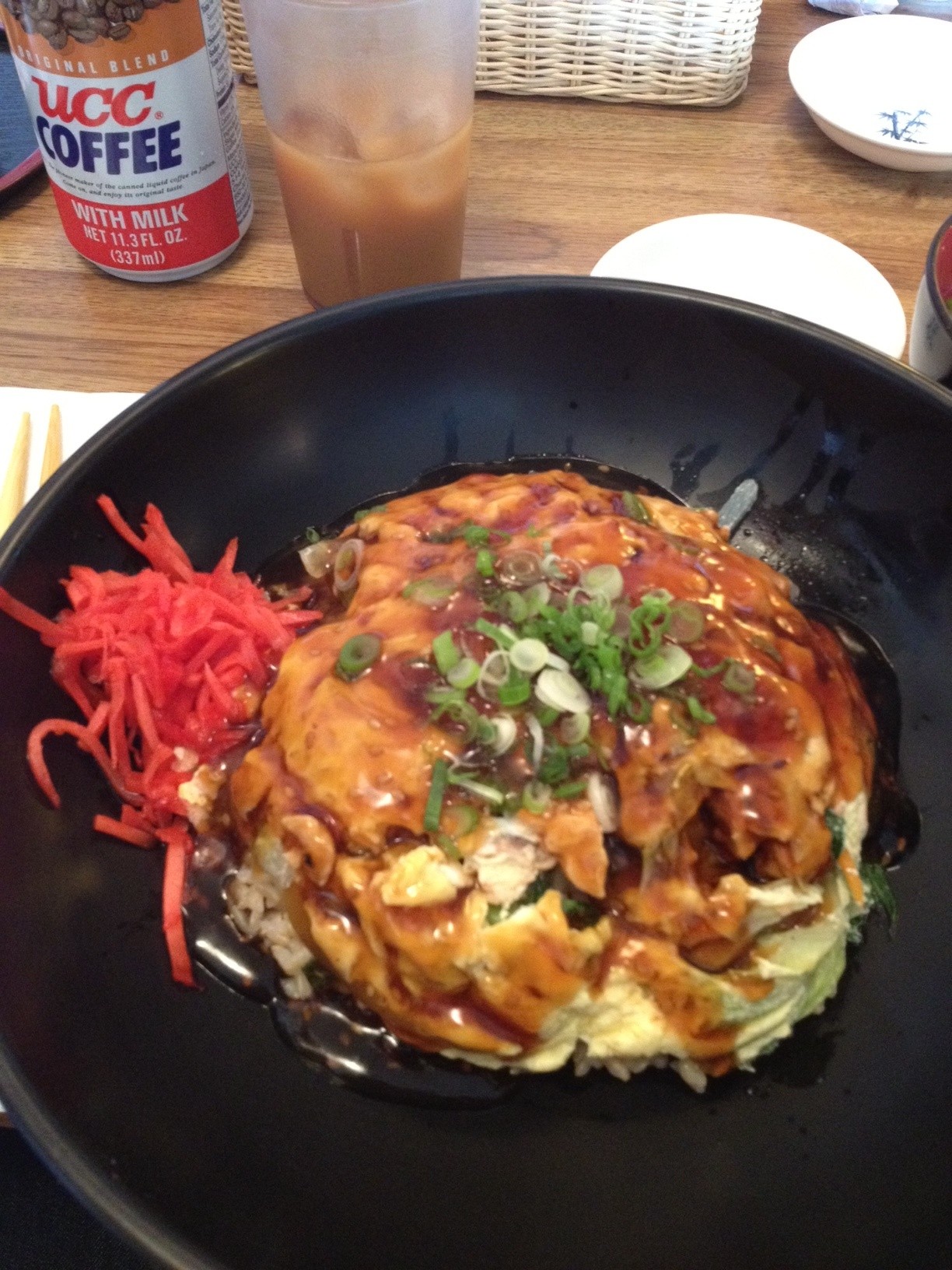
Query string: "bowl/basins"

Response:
xmin=786 ymin=13 xmax=951 ymax=177
xmin=0 ymin=278 xmax=952 ymax=1270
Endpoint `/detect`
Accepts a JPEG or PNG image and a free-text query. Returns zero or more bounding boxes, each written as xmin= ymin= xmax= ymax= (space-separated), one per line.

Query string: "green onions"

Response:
xmin=303 ymin=492 xmax=759 ymax=858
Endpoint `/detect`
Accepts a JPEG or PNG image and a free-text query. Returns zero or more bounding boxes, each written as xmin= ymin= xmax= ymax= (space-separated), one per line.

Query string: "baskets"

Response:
xmin=222 ymin=0 xmax=762 ymax=107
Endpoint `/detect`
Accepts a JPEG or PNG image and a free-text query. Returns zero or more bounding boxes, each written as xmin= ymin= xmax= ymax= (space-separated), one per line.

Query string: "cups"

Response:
xmin=906 ymin=214 xmax=951 ymax=386
xmin=234 ymin=1 xmax=477 ymax=316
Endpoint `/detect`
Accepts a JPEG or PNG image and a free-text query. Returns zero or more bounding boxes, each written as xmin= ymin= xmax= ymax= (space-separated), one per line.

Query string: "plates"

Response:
xmin=588 ymin=213 xmax=908 ymax=365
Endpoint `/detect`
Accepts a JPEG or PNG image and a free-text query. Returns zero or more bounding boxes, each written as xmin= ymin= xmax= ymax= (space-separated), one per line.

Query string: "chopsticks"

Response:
xmin=0 ymin=404 xmax=60 ymax=537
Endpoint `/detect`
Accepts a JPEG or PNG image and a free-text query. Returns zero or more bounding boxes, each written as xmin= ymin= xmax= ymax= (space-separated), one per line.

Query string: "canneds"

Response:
xmin=0 ymin=0 xmax=255 ymax=285
